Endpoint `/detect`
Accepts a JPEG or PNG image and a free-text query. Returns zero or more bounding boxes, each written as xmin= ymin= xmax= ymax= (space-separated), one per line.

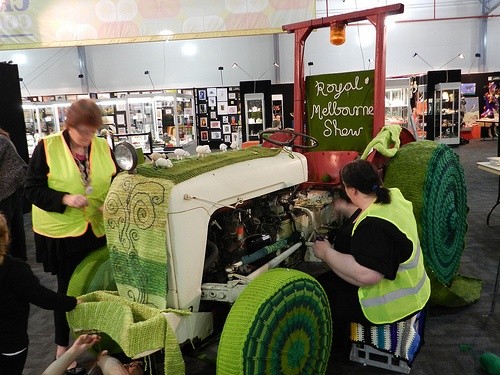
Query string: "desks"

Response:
xmin=476 ymin=119 xmax=499 ymax=141
xmin=477 ymin=162 xmax=500 ymax=225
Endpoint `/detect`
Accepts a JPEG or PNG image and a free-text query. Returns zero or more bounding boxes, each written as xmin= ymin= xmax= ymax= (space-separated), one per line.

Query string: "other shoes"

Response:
xmin=54 ymin=356 xmax=84 ymax=375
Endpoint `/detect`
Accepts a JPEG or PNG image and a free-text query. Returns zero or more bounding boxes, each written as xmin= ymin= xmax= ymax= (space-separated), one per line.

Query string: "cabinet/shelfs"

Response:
xmin=434 ymin=82 xmax=461 ymax=145
xmin=244 ymin=93 xmax=265 ymax=142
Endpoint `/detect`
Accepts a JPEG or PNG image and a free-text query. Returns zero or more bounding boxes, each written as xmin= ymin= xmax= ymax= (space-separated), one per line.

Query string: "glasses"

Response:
xmin=72 ymin=125 xmax=96 ymax=135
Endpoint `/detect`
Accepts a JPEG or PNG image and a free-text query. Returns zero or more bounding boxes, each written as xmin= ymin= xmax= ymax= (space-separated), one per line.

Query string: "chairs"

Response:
xmin=303 ymin=151 xmax=359 ymax=191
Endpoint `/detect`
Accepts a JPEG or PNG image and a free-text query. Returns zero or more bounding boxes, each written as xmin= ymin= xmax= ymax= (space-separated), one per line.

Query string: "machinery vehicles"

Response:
xmin=66 ymin=2 xmax=469 ymax=375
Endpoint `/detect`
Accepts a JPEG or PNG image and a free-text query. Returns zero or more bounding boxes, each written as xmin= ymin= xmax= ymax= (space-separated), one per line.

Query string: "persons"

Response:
xmin=40 ymin=333 xmax=145 ymax=375
xmin=313 ymin=159 xmax=431 ymax=375
xmin=0 ymin=129 xmax=29 ymax=264
xmin=480 ymin=82 xmax=498 ymax=138
xmin=0 ymin=210 xmax=83 ymax=375
xmin=26 ymin=99 xmax=122 ymax=375
